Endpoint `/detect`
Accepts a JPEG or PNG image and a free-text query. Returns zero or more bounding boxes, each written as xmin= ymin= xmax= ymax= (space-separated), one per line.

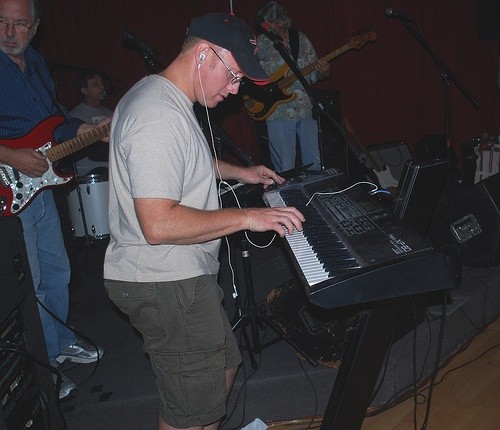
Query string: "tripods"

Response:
xmin=227 ymin=232 xmax=316 ymax=369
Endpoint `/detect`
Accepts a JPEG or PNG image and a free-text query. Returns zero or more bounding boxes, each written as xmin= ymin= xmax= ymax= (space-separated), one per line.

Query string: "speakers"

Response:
xmin=261 ymin=273 xmax=424 ymax=366
xmin=431 ymin=172 xmax=500 ymax=267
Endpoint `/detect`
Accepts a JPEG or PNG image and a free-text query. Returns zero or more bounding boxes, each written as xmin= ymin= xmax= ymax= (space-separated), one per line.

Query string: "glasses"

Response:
xmin=209 ymin=46 xmax=245 ymax=89
xmin=0 ymin=17 xmax=34 ymax=33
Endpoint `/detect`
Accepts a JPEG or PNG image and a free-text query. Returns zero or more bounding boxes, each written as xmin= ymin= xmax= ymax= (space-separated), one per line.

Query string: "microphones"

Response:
xmin=121 ymin=29 xmax=155 ymax=55
xmin=385 ymin=9 xmax=416 ymax=22
xmin=255 ymin=16 xmax=283 ymax=40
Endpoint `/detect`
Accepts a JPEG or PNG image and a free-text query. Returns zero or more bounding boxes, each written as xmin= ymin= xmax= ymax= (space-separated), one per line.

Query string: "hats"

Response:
xmin=188 ymin=13 xmax=272 ymax=86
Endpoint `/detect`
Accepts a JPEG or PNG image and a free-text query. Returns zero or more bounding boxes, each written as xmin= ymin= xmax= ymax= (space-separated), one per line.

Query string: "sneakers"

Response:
xmin=49 ymin=335 xmax=104 ymax=368
xmin=52 ymin=370 xmax=78 ymax=402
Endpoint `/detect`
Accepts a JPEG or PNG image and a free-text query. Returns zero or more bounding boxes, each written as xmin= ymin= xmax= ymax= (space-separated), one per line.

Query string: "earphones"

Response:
xmin=200 ymin=54 xmax=206 ymax=61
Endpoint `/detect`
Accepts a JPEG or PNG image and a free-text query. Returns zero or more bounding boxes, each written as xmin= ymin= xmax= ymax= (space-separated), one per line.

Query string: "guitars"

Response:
xmin=241 ymin=30 xmax=378 ymax=122
xmin=0 ymin=115 xmax=120 ymax=217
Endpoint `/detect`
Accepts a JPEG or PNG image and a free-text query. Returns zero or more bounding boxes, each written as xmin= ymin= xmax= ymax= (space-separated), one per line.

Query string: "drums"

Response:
xmin=61 ymin=174 xmax=117 ymax=241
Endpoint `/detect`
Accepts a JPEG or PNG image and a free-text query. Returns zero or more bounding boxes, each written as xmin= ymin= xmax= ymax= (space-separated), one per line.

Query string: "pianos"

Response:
xmin=259 ymin=166 xmax=464 ymax=430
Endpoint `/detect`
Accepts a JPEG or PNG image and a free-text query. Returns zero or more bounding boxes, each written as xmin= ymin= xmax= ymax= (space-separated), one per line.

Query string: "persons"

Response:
xmin=68 ymin=70 xmax=113 ymax=125
xmin=103 ymin=11 xmax=306 ymax=430
xmin=252 ymin=2 xmax=331 ymax=172
xmin=0 ymin=0 xmax=112 ymax=401
